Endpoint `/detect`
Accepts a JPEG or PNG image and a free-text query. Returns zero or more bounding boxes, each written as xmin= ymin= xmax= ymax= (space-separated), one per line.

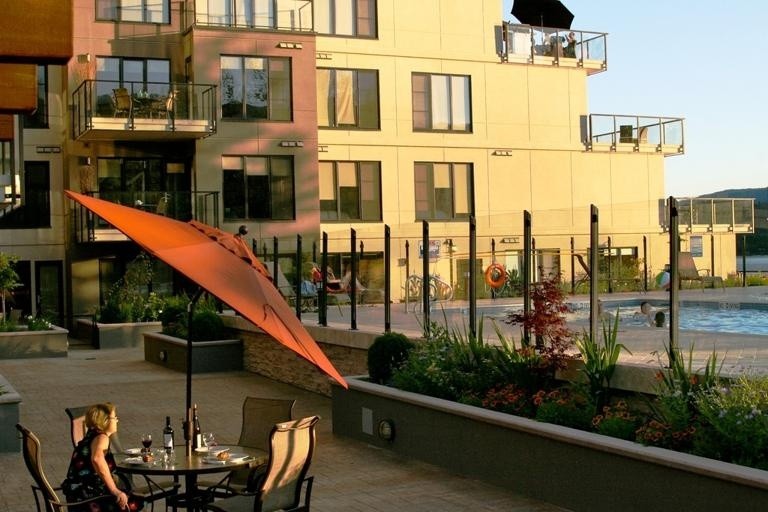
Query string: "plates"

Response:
xmin=194 ymin=445 xmax=230 ymax=455
xmin=121 ymin=456 xmax=163 ymax=465
xmin=124 ymin=447 xmax=160 ymax=455
xmin=202 ymin=452 xmax=250 ymax=464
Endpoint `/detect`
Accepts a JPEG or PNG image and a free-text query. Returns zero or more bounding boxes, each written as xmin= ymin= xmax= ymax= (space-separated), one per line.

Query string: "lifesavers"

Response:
xmin=486 ymin=264 xmax=505 ymax=287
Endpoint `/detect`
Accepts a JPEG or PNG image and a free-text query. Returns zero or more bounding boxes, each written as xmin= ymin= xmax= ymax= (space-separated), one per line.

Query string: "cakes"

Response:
xmin=217 ymin=451 xmax=230 ymax=461
xmin=142 ymin=455 xmax=151 ymax=462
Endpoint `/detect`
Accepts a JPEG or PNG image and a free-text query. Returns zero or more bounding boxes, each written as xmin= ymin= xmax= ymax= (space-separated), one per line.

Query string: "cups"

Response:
xmin=141 ymin=433 xmax=152 ymax=448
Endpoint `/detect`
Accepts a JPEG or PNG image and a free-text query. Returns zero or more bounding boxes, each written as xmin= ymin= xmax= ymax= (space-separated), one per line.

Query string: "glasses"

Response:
xmin=106 ymin=415 xmax=118 ymax=421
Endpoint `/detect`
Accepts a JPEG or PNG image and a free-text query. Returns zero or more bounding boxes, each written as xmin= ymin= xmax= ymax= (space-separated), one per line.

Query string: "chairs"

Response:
xmin=261 ymin=260 xmax=344 ymax=318
xmin=677 ymin=251 xmax=727 ymax=294
xmin=112 ymin=88 xmax=179 ymax=119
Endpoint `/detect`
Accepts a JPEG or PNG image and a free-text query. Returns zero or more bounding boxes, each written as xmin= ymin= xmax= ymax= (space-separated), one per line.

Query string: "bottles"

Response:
xmin=163 ymin=415 xmax=175 ymax=454
xmin=192 ymin=403 xmax=202 ymax=452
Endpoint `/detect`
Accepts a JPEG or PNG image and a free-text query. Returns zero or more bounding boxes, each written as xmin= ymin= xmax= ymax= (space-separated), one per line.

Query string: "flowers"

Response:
xmin=480 ymin=375 xmax=768 ymax=451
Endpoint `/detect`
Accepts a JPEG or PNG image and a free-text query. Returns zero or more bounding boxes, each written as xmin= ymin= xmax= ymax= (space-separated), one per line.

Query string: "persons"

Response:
xmin=635 ymin=301 xmax=653 ymax=315
xmin=324 ymin=265 xmax=368 ymax=292
xmin=562 ymin=31 xmax=576 ymax=58
xmin=597 ymin=299 xmax=611 ymax=321
xmin=60 ymin=402 xmax=148 ymax=512
xmin=647 ymin=310 xmax=667 ymax=327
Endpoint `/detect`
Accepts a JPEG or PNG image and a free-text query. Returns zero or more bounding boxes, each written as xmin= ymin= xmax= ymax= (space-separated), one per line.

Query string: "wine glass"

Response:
xmin=202 ymin=432 xmax=215 ymax=451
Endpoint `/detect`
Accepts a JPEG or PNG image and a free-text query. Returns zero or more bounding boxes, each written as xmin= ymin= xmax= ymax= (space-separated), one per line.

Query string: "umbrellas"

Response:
xmin=511 ymin=0 xmax=577 ymax=47
xmin=62 ymin=187 xmax=350 ymax=451
